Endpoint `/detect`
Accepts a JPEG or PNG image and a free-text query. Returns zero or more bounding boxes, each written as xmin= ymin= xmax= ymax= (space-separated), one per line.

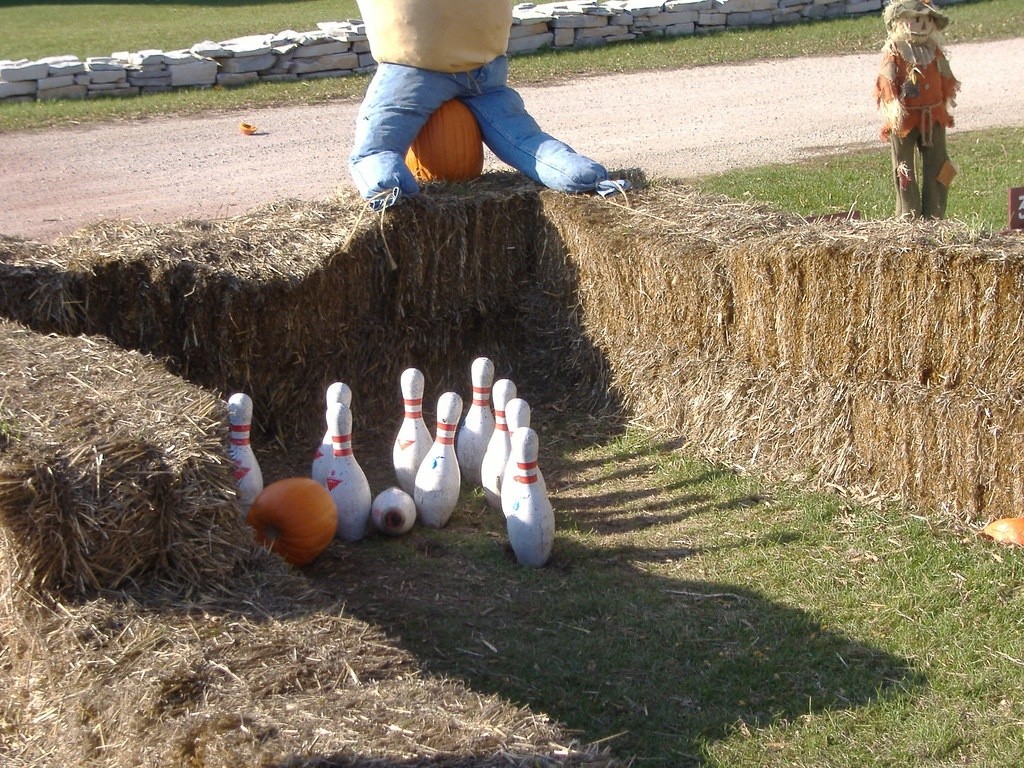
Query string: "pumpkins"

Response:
xmin=404 ymin=97 xmax=483 ymax=182
xmin=983 ymin=517 xmax=1024 ymax=546
xmin=248 ymin=479 xmax=337 ymax=564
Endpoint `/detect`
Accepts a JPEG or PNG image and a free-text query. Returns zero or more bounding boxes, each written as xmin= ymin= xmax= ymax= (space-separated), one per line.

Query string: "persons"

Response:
xmin=348 ymin=0 xmax=632 ymax=213
xmin=878 ymin=0 xmax=961 ymax=219
